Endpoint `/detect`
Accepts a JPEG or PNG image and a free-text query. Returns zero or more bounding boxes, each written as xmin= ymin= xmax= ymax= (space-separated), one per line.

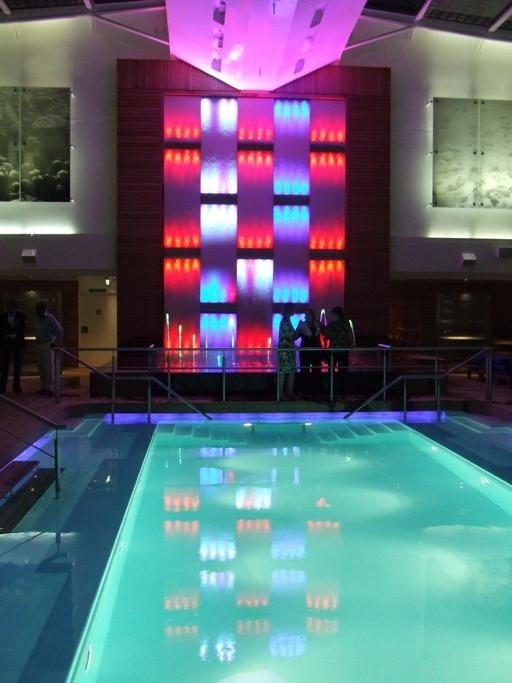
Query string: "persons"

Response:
xmin=318 ymin=305 xmax=354 ymax=398
xmin=298 ymin=308 xmax=323 ymax=399
xmin=0 ymin=297 xmax=27 ymax=394
xmin=31 ymin=302 xmax=65 ymax=397
xmin=277 ymin=302 xmax=301 ymax=402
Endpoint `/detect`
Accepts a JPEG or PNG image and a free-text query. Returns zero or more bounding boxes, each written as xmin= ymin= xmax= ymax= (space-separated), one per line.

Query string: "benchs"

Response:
xmin=391 ymin=333 xmax=511 ymax=405
